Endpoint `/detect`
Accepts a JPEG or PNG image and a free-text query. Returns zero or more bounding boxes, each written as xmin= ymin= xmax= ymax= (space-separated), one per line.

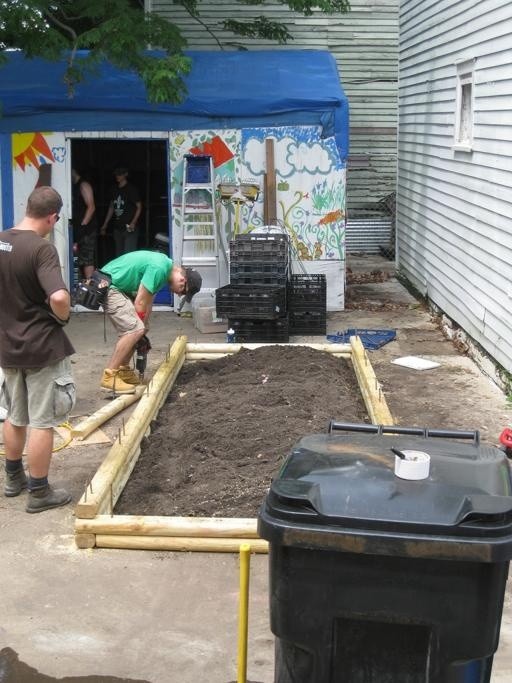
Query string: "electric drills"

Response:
xmin=136 ymin=349 xmax=148 ymax=385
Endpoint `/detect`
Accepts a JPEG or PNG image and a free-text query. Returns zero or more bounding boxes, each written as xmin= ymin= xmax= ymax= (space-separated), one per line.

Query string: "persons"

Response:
xmin=100 ymin=167 xmax=142 ymax=257
xmin=71 ymin=167 xmax=98 ymax=279
xmin=0 ymin=186 xmax=77 ymax=513
xmin=97 ymin=250 xmax=202 ymax=394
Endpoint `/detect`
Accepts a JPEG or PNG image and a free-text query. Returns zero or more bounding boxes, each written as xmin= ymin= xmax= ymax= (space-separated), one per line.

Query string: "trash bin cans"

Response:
xmin=258 ymin=420 xmax=511 ymax=683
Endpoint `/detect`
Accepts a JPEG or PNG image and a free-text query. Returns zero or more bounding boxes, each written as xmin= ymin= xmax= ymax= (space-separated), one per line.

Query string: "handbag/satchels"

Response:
xmin=76 ymin=269 xmax=112 ymax=310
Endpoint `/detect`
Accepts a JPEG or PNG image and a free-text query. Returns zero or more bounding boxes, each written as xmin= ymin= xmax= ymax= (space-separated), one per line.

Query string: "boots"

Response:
xmin=3 ymin=466 xmax=28 ymax=497
xmin=26 ymin=484 xmax=71 ymax=513
xmin=100 ymin=365 xmax=140 ymax=393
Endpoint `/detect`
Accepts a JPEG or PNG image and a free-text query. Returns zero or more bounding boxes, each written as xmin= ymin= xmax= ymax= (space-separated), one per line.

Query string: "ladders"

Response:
xmin=177 ymin=154 xmax=230 ymax=316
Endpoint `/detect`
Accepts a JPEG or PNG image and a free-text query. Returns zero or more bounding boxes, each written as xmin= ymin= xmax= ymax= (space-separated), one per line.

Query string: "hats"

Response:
xmin=113 ymin=168 xmax=128 ymax=175
xmin=185 ymin=268 xmax=203 ymax=304
